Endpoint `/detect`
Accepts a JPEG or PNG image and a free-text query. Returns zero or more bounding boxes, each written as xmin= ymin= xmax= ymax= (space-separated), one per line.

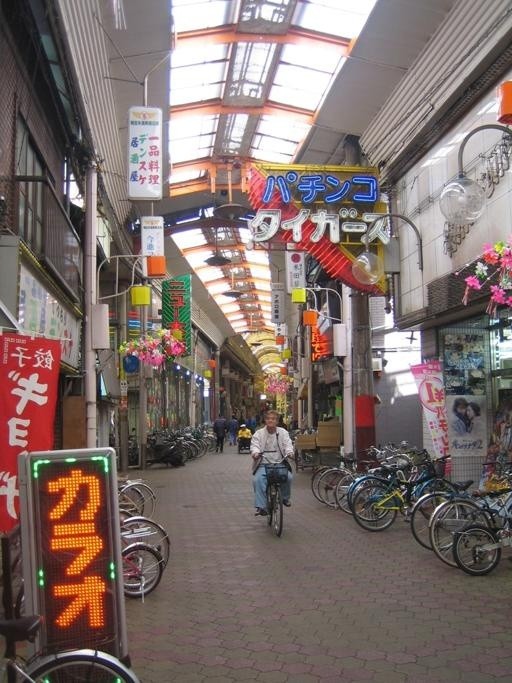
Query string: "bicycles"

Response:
xmin=285 ymin=425 xmax=317 ymax=466
xmin=312 ymin=438 xmax=512 ymax=575
xmin=108 ymin=422 xmax=217 ymax=467
xmin=6 ymin=473 xmax=169 ymax=618
xmin=258 ymin=452 xmax=294 ymax=537
xmin=1 ymin=614 xmax=139 ymax=683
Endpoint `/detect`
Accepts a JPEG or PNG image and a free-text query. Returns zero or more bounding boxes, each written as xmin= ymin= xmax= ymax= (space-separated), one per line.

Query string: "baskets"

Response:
xmin=264 ymin=463 xmax=289 ymax=484
xmin=432 ymin=460 xmax=445 ymax=477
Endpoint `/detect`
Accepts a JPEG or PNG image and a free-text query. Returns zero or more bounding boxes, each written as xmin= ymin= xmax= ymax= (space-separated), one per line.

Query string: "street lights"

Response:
xmin=131 ymin=283 xmax=151 ymax=468
xmin=292 ymin=286 xmax=318 ymax=430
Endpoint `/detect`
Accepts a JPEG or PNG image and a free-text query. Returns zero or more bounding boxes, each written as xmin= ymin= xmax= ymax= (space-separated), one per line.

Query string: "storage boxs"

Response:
xmin=316 ymin=420 xmax=342 ymax=448
xmin=293 ymin=432 xmax=315 ymax=449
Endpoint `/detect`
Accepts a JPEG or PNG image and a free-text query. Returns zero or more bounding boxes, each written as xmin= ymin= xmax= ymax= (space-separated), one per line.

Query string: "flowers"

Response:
xmin=96 ymin=326 xmax=185 ymax=373
xmin=455 ymin=231 xmax=512 ymax=319
xmin=264 ymin=373 xmax=308 ymax=393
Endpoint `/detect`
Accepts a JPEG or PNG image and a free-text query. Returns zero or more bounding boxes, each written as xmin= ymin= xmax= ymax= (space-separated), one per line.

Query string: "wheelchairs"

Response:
xmin=237 ymin=436 xmax=252 ymax=453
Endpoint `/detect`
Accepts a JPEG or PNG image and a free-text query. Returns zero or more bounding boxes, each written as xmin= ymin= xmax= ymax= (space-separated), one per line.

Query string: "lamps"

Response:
xmin=203 ymin=159 xmax=264 ymax=348
xmin=438 ymin=123 xmax=512 ymax=226
xmin=352 ymin=213 xmax=425 ymax=286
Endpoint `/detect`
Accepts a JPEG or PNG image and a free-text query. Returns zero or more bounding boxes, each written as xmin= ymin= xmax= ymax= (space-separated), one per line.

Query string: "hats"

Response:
xmin=240 ymin=424 xmax=246 ymax=428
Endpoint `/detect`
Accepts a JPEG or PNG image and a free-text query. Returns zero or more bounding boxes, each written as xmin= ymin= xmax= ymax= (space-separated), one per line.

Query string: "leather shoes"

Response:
xmin=254 ymin=507 xmax=266 ymax=515
xmin=278 ymin=497 xmax=291 ymax=506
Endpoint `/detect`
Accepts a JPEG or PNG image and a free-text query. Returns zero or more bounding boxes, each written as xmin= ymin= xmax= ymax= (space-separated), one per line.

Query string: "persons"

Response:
xmin=250 ymin=410 xmax=295 ymax=517
xmin=214 ymin=413 xmax=262 ymax=453
xmin=447 ymin=395 xmax=489 ymax=451
